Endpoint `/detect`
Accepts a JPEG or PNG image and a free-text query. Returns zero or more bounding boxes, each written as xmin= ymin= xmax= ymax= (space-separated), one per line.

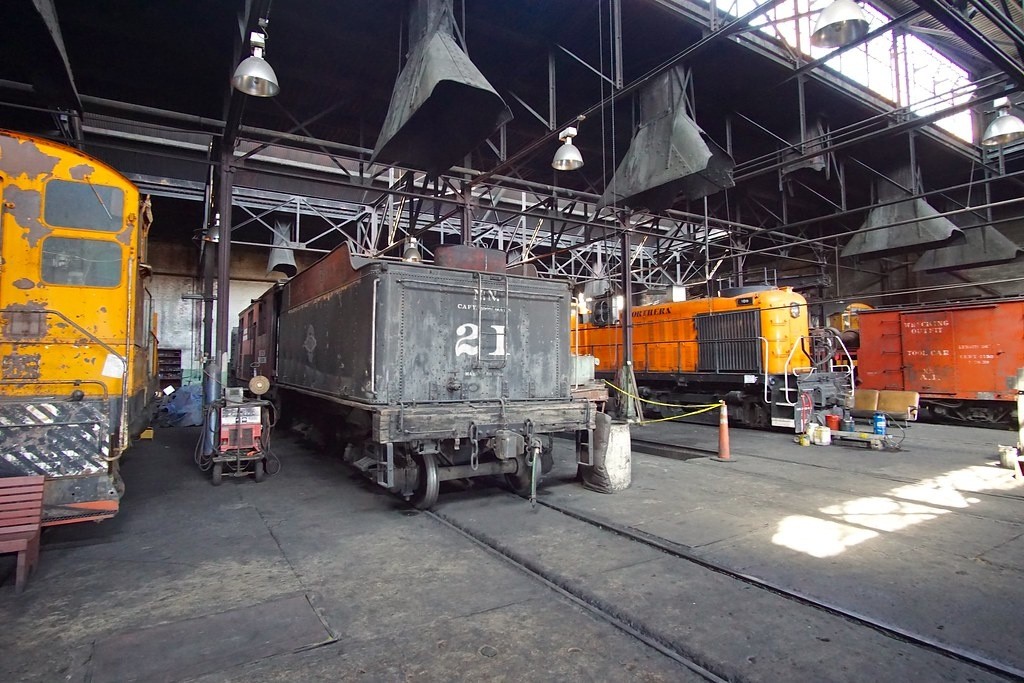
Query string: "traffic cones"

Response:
xmin=718 ymin=401 xmax=731 ymax=458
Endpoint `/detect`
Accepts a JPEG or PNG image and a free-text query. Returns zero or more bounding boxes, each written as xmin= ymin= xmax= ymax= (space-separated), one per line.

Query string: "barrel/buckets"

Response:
xmin=825 ymin=414 xmax=854 ymax=432
xmin=793 ymin=422 xmax=831 ymax=447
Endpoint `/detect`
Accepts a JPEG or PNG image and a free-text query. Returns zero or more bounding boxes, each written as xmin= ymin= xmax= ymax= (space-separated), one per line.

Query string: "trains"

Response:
xmin=228 ymin=236 xmax=1024 ymax=510
xmin=0 ymin=128 xmax=161 ymax=537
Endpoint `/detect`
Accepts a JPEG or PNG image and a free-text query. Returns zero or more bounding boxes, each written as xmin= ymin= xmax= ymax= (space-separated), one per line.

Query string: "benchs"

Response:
xmin=0 ymin=475 xmax=45 ymax=592
xmin=842 ymin=390 xmax=920 ymax=452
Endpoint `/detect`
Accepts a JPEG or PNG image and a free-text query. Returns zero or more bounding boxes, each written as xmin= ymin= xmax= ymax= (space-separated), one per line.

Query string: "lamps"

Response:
xmin=403 ymin=237 xmax=421 ymax=262
xmin=234 ymin=32 xmax=278 ymax=98
xmin=981 ymin=95 xmax=1024 ymax=146
xmin=552 ymin=127 xmax=583 ymax=169
xmin=204 ymin=213 xmax=221 ymax=243
xmin=810 ymin=0 xmax=871 ymax=48
